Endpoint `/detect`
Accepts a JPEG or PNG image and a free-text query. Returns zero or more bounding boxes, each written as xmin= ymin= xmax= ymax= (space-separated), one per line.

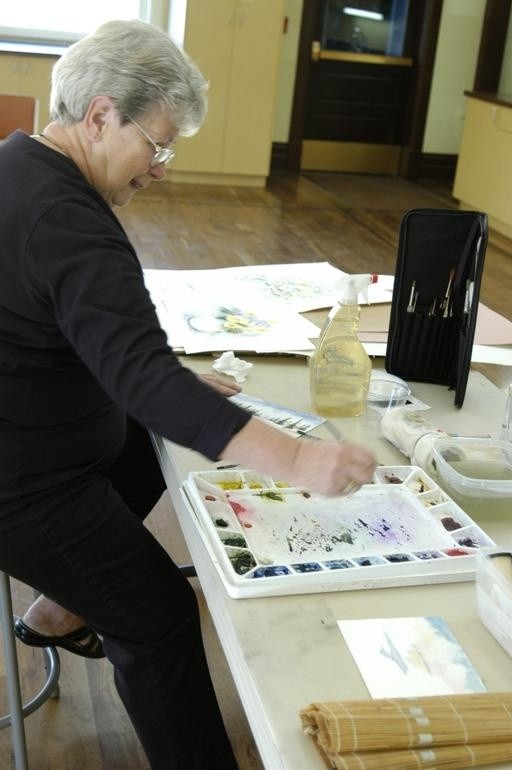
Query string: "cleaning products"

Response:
xmin=310 ymin=272 xmax=379 ymax=415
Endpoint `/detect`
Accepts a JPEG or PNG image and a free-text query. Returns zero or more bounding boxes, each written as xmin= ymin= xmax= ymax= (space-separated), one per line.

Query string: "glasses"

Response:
xmin=127 ymin=114 xmax=176 ymax=168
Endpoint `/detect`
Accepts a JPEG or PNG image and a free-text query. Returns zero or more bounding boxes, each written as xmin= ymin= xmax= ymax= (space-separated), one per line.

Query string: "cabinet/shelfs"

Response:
xmin=165 ymin=0 xmax=305 ymax=194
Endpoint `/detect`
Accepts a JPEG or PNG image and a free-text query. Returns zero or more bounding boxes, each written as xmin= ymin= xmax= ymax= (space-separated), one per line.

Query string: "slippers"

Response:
xmin=13 ymin=618 xmax=106 ymax=658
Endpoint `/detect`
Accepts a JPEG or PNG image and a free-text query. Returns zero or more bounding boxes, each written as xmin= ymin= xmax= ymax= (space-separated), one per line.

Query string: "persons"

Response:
xmin=1 ymin=18 xmax=380 ymax=767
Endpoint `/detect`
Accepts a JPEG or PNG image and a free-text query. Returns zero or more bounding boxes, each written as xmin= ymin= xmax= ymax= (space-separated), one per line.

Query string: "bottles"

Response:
xmin=380 ymin=405 xmax=467 ymax=478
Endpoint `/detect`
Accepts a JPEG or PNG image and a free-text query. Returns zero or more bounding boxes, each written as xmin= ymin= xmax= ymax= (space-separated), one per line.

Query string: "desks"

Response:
xmin=142 ymin=267 xmax=509 ymax=768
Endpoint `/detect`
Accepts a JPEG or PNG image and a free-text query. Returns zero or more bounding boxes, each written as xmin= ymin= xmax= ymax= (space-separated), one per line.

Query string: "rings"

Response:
xmin=340 ymin=478 xmax=358 ymax=497
